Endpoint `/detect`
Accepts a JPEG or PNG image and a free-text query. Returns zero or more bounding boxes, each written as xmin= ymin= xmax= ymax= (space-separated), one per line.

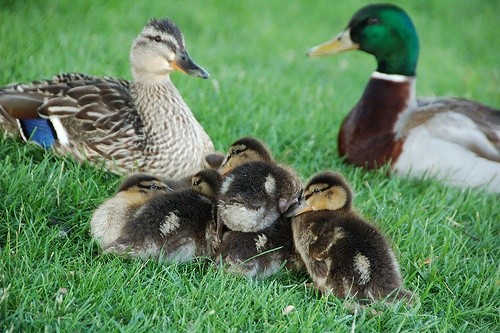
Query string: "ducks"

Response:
xmin=1 ymin=17 xmax=218 ymax=179
xmin=306 ymin=2 xmax=500 ymax=192
xmin=283 ymin=170 xmax=417 ymax=313
xmin=90 ymin=137 xmax=308 ymax=280
